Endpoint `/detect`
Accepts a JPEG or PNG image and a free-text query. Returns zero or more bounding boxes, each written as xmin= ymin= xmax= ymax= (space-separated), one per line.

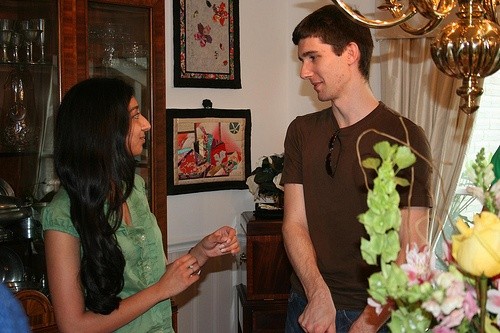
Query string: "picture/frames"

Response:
xmin=164 ymin=108 xmax=252 ymax=195
xmin=172 ymin=0 xmax=243 ymax=87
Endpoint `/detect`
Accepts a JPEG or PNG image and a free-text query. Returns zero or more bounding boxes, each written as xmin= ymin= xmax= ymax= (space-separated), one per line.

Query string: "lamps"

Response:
xmin=331 ymin=0 xmax=500 ymax=118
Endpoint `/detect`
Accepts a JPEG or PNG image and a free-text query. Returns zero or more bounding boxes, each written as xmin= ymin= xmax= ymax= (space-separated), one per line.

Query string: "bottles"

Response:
xmin=1 ymin=70 xmax=39 ymax=152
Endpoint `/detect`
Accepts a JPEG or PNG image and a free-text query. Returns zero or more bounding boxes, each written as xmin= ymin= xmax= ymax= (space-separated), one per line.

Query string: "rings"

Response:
xmin=189 ymin=266 xmax=195 ymax=273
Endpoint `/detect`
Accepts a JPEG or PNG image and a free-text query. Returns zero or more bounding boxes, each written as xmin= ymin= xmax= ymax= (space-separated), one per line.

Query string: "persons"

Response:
xmin=279 ymin=3 xmax=434 ymax=333
xmin=38 ymin=77 xmax=241 ymax=333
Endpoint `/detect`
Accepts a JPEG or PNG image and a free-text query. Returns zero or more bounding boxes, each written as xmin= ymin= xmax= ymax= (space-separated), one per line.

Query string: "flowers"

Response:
xmin=356 ymin=117 xmax=500 ymax=333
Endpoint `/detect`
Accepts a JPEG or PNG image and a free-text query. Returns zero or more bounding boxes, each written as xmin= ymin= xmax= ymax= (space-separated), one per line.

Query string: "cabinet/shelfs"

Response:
xmin=0 ymin=1 xmax=63 ymax=308
xmin=55 ymin=1 xmax=169 ymax=264
xmin=236 ymin=211 xmax=293 ymax=333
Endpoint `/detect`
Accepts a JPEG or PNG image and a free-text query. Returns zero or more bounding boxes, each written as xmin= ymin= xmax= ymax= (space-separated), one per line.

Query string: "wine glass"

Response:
xmin=0 ymin=15 xmax=51 ymax=67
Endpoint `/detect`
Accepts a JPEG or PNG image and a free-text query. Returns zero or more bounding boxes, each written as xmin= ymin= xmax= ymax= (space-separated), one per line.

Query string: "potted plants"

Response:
xmin=246 ymin=151 xmax=287 ymax=219
